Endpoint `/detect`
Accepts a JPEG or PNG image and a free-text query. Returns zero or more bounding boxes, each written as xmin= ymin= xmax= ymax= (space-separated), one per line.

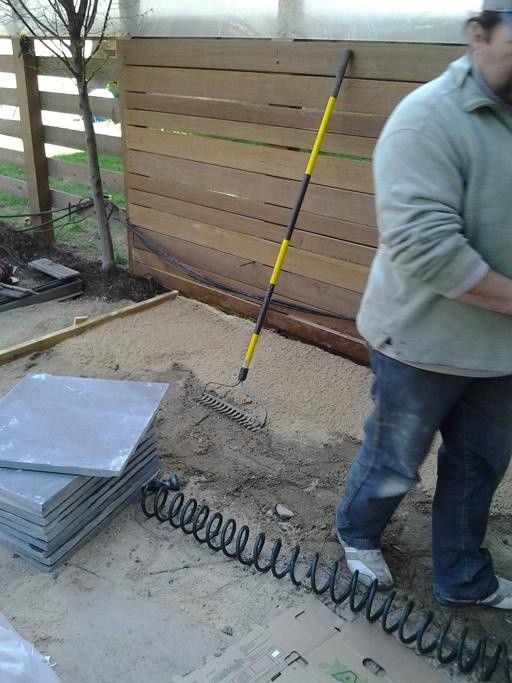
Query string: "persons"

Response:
xmin=333 ymin=0 xmax=512 ymax=610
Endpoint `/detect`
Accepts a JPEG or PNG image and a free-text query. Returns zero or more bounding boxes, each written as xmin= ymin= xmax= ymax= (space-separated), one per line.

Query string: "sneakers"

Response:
xmin=335 ymin=528 xmax=393 ymax=589
xmin=435 ymin=575 xmax=512 ymax=610
